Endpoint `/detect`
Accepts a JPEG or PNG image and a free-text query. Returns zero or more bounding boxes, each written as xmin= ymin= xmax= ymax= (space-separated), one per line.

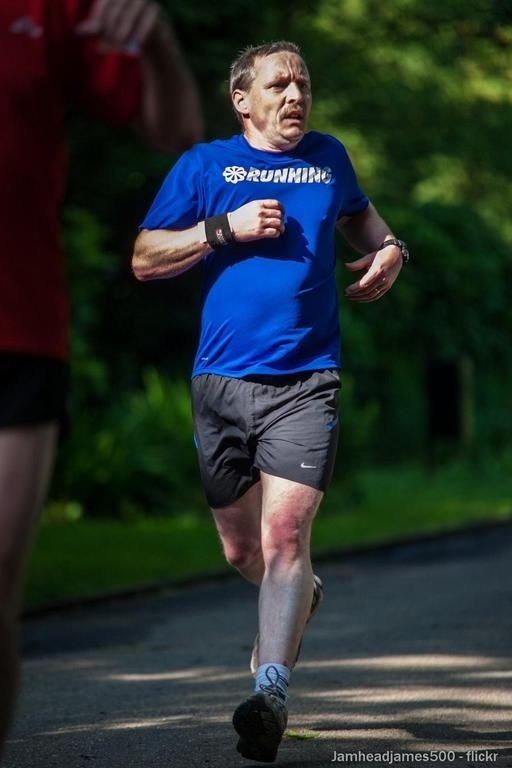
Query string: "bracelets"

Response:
xmin=204 ymin=213 xmax=236 ymax=250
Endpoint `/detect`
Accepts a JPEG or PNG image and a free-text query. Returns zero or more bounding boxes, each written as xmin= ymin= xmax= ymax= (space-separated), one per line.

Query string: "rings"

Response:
xmin=375 ymin=289 xmax=380 ymax=293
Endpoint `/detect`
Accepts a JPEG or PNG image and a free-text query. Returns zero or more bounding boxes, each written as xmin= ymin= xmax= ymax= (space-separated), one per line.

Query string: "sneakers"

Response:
xmin=233 ymin=684 xmax=289 ymax=763
xmin=250 ymin=574 xmax=324 ymax=680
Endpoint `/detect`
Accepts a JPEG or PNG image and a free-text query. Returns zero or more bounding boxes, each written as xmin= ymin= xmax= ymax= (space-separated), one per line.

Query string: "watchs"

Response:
xmin=379 ymin=238 xmax=410 ymax=265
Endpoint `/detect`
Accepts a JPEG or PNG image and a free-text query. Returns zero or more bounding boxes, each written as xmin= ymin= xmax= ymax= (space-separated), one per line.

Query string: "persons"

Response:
xmin=0 ymin=0 xmax=204 ymax=749
xmin=131 ymin=41 xmax=409 ymax=763
